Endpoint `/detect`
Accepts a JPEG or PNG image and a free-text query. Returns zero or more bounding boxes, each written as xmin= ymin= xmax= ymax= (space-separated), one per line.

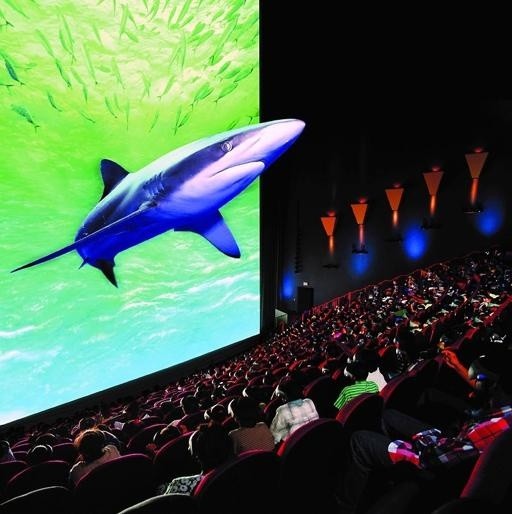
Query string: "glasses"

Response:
xmin=320 ymin=151 xmax=488 ymax=236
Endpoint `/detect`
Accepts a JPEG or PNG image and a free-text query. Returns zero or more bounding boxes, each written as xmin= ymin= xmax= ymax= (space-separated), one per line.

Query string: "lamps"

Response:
xmin=320 ymin=151 xmax=488 ymax=236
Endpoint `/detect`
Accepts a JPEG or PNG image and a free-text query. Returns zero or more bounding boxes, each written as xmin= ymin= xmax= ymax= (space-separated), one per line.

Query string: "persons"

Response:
xmin=0 ymin=244 xmax=512 ymax=514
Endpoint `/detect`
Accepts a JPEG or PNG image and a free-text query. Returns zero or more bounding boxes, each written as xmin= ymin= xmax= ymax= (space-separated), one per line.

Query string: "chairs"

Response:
xmin=2 ymin=249 xmax=512 ymax=513
